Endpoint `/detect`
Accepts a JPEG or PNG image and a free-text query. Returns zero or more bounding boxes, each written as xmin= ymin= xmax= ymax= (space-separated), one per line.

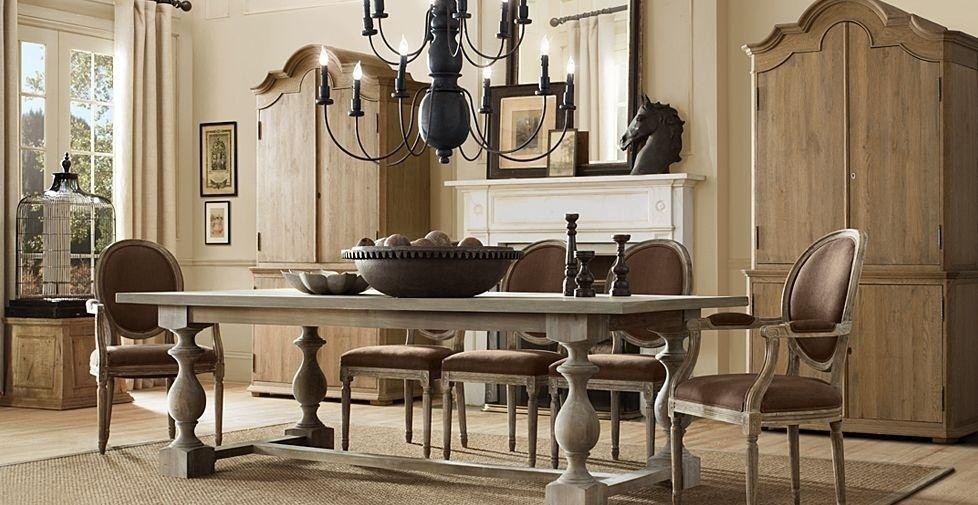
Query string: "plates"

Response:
xmin=280 ymin=268 xmax=372 ymax=294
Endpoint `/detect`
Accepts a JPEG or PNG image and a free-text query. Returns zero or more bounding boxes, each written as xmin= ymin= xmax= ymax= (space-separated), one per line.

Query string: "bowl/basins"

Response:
xmin=340 ymin=245 xmax=524 ymax=297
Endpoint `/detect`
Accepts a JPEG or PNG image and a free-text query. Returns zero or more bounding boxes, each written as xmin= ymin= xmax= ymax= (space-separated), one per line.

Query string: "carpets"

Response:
xmin=0 ymin=419 xmax=954 ymax=505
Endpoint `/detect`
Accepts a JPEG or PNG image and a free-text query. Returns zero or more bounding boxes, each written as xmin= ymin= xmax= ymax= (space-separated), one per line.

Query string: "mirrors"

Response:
xmin=505 ymin=0 xmax=639 ymax=175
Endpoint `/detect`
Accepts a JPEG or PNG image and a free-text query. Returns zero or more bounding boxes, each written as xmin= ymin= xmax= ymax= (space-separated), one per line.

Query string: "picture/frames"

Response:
xmin=203 ymin=200 xmax=230 ymax=245
xmin=199 ymin=121 xmax=238 ymax=197
xmin=576 ymin=130 xmax=589 ymax=164
xmin=485 ymin=81 xmax=575 ymax=179
xmin=546 ymin=128 xmax=578 ymax=177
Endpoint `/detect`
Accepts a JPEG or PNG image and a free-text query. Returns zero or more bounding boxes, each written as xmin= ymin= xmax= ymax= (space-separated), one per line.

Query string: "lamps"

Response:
xmin=318 ymin=0 xmax=576 ymax=167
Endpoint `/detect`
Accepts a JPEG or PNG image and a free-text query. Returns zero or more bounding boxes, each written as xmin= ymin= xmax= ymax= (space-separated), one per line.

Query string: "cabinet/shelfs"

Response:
xmin=745 ymin=0 xmax=978 ymax=442
xmin=8 ymin=319 xmax=135 ymax=410
xmin=250 ymin=43 xmax=434 ymax=404
